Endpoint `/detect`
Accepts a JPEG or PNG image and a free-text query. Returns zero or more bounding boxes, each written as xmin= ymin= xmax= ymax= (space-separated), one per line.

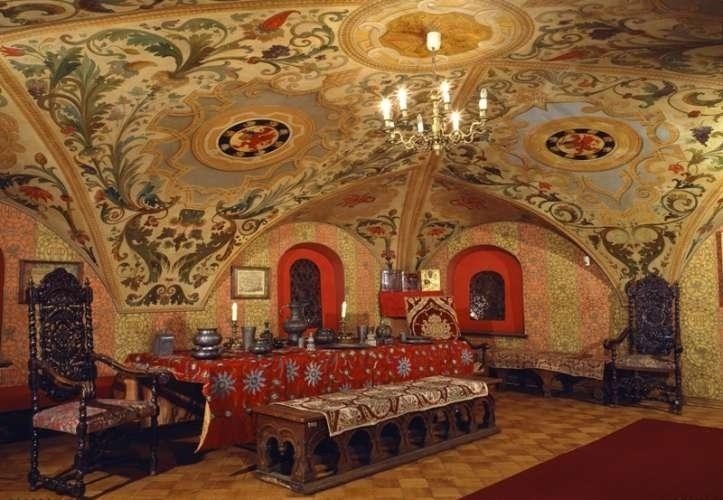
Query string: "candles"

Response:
xmin=340 ymin=299 xmax=348 ymax=317
xmin=231 ymin=301 xmax=238 ymax=321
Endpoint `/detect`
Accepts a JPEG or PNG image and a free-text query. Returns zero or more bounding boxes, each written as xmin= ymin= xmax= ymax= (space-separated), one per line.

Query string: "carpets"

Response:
xmin=459 ymin=417 xmax=722 ymax=500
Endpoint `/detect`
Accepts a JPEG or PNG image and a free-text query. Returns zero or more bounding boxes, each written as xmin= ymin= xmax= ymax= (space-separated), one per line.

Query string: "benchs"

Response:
xmin=250 ymin=372 xmax=502 ymax=498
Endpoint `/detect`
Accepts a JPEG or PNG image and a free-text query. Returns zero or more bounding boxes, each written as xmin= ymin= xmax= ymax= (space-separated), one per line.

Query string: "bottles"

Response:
xmin=381 ymin=270 xmax=416 ymax=292
xmin=317 ymin=327 xmax=335 ymax=343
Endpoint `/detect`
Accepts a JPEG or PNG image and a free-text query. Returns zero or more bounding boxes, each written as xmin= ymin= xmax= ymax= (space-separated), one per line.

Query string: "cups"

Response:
xmin=356 ymin=324 xmax=367 ymax=343
xmin=400 ymin=332 xmax=405 ymax=342
xmin=240 ymin=326 xmax=256 ymax=345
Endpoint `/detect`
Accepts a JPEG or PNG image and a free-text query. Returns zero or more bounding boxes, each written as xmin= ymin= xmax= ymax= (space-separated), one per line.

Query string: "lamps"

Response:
xmin=377 ymin=30 xmax=492 ymax=161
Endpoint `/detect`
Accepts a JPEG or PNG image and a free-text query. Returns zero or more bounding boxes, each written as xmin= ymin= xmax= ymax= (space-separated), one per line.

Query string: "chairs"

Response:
xmin=603 ymin=271 xmax=685 ymax=414
xmin=403 ymin=294 xmax=492 ymax=375
xmin=21 ymin=265 xmax=172 ymax=498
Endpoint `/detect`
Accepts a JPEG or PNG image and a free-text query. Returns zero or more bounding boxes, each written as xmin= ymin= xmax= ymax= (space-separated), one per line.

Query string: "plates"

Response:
xmin=405 ymin=336 xmax=431 ymax=344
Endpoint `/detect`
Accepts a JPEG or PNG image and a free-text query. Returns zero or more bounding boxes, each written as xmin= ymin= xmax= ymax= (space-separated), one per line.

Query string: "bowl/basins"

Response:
xmin=249 ymin=345 xmax=271 ymax=355
xmin=191 ymin=350 xmax=218 ymax=360
xmin=375 ymin=325 xmax=391 ymax=336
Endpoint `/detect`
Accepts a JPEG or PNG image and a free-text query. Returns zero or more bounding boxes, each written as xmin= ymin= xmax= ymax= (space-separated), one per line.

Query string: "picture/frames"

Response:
xmin=229 ymin=263 xmax=273 ymax=300
xmin=18 ymin=257 xmax=85 ymax=305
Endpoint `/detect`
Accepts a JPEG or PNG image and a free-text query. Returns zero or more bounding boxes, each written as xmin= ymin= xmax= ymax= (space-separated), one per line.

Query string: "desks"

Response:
xmin=484 ymin=346 xmax=606 ymax=398
xmin=119 ymin=335 xmax=484 ymax=479
